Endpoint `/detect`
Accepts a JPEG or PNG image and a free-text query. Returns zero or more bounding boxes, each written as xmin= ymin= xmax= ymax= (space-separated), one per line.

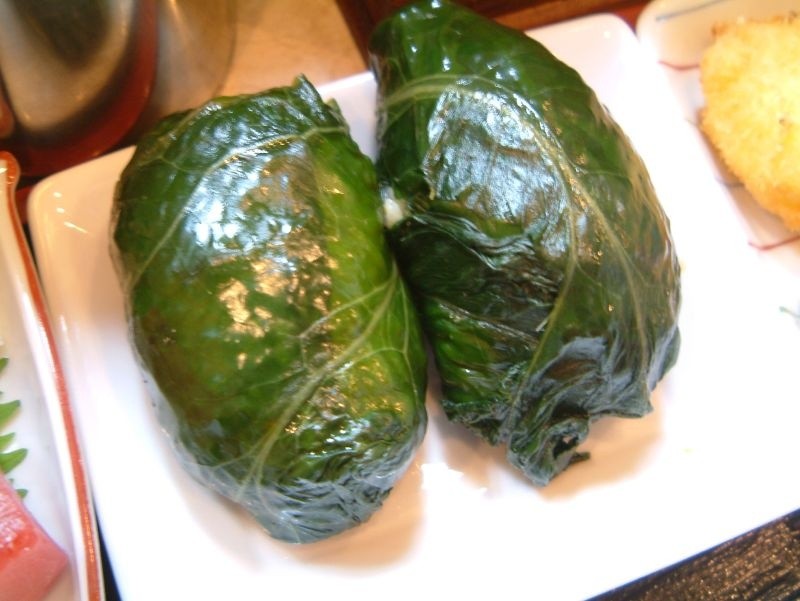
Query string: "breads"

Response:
xmin=699 ymin=7 xmax=799 ymax=243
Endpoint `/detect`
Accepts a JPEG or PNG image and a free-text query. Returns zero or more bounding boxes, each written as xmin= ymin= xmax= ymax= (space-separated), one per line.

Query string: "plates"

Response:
xmin=0 ymin=149 xmax=108 ymax=601
xmin=640 ymin=0 xmax=800 ymax=352
xmin=27 ymin=11 xmax=799 ymax=600
